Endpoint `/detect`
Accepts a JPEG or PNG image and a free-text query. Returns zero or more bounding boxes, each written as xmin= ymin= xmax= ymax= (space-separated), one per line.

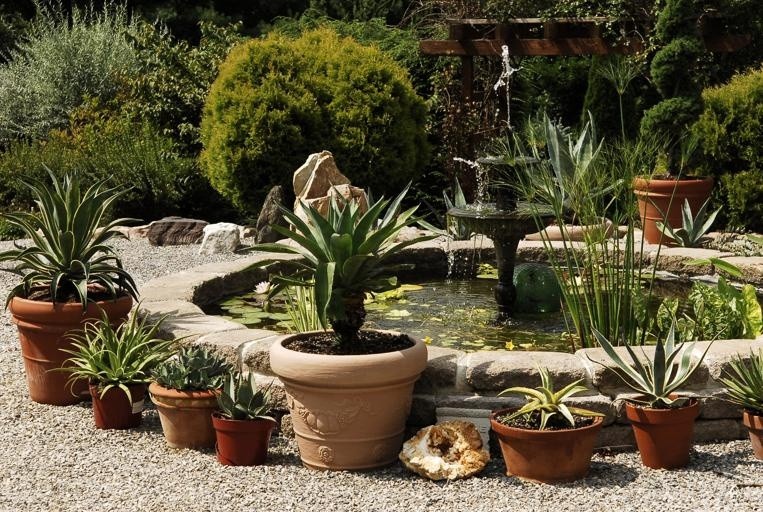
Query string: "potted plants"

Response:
xmin=717 ymin=348 xmax=762 ymax=461
xmin=634 ymin=149 xmax=714 ymax=245
xmin=2 ymin=161 xmax=139 ymax=408
xmin=149 ymin=341 xmax=229 ymax=451
xmin=585 ymin=315 xmax=723 ymax=470
xmin=209 ymin=369 xmax=283 ymax=466
xmin=256 ymin=180 xmax=430 ymax=474
xmin=64 ymin=301 xmax=177 ymax=431
xmin=490 ymin=360 xmax=600 ymax=484
